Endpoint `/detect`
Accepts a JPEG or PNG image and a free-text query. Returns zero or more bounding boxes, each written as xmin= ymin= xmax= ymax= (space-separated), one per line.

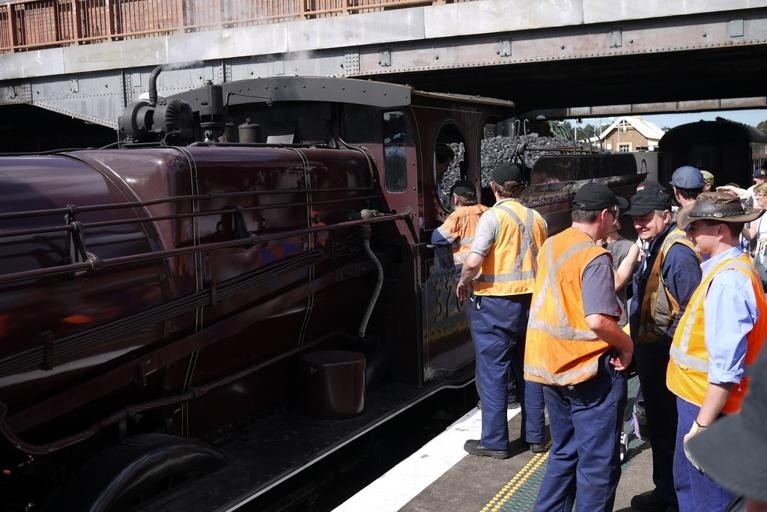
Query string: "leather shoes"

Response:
xmin=630 ymin=490 xmax=679 ymax=510
xmin=463 ymin=438 xmax=511 ymax=460
xmin=529 ymin=438 xmax=547 ymax=453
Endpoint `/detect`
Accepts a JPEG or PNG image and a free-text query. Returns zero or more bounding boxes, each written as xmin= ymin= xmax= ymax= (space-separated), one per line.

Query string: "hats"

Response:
xmin=668 ymin=165 xmax=705 ymax=189
xmin=573 ymin=182 xmax=629 ymax=210
xmin=622 ymin=187 xmax=672 ymax=215
xmin=450 ymin=179 xmax=476 ymax=197
xmin=674 ymin=194 xmax=766 ymax=230
xmin=491 ymin=162 xmax=525 ymax=188
xmin=685 ymin=337 xmax=766 ymax=507
xmin=753 ymin=168 xmax=767 ymax=179
xmin=699 ymin=169 xmax=715 ymax=185
xmin=715 ymin=185 xmax=754 ymax=208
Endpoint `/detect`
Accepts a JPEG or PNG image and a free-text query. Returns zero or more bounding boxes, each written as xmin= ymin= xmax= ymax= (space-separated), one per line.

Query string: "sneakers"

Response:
xmin=476 ymin=400 xmax=520 ymax=410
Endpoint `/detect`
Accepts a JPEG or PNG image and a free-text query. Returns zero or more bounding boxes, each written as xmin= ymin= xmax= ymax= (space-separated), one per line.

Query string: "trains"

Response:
xmin=0 ymin=60 xmax=767 ymax=512
